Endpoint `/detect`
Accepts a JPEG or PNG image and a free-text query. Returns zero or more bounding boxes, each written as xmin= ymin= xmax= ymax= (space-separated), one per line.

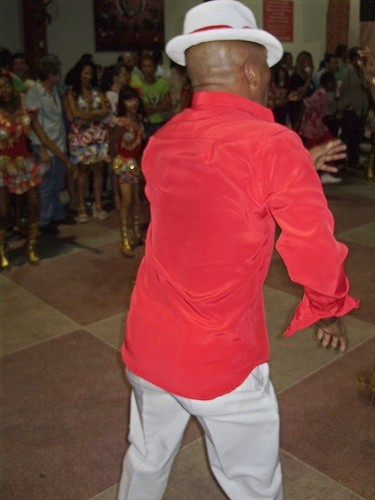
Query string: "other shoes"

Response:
xmin=90 ymin=191 xmax=110 ymax=199
xmin=320 ymin=171 xmax=341 ymax=188
xmin=38 ymin=225 xmax=60 ymax=236
xmin=51 ymin=216 xmax=78 ymax=225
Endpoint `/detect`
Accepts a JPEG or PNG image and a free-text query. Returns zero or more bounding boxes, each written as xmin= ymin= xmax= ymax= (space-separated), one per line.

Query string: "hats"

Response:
xmin=166 ymin=0 xmax=284 ymax=69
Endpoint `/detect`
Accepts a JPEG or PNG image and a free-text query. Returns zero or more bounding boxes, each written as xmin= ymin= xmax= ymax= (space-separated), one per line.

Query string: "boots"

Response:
xmin=119 ymin=215 xmax=135 ymax=257
xmin=365 ymin=154 xmax=374 ymax=178
xmin=27 ymin=220 xmax=41 ymax=265
xmin=133 ymin=214 xmax=145 ymax=243
xmin=0 ymin=228 xmax=9 ymax=270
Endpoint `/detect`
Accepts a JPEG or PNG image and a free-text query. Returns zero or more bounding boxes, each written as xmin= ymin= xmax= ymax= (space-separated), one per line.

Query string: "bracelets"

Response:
xmin=153 ymin=104 xmax=157 ymax=113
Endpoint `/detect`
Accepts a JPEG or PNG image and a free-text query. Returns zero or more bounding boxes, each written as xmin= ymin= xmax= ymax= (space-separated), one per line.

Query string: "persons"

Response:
xmin=268 ymin=45 xmax=375 ymax=183
xmin=0 ymin=47 xmax=190 ymax=268
xmin=112 ymin=0 xmax=361 ymax=500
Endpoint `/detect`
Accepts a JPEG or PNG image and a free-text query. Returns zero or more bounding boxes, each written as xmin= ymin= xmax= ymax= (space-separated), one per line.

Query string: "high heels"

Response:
xmin=76 ymin=205 xmax=89 ymax=224
xmin=91 ymin=202 xmax=109 ymax=221
